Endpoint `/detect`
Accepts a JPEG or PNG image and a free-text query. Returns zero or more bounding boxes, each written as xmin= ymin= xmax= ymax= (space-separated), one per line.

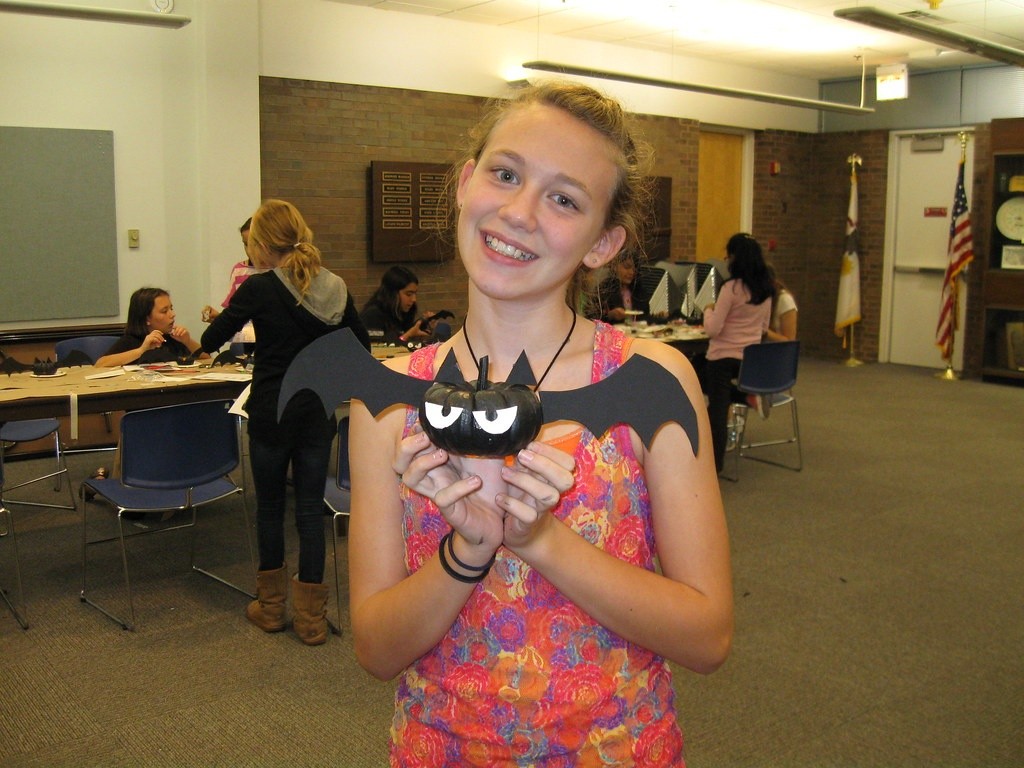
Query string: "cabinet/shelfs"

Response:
xmin=978 ymin=150 xmax=1024 ymax=384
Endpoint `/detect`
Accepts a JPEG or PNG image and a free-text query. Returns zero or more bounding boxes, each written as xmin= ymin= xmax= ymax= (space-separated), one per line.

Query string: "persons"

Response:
xmin=202 ymin=217 xmax=271 ymax=358
xmin=357 ymin=266 xmax=438 ymax=348
xmin=201 ymin=200 xmax=371 ymax=646
xmin=79 ymin=287 xmax=212 ymax=523
xmin=692 ymin=233 xmax=773 ymax=477
xmin=348 ymin=84 xmax=735 ymax=768
xmin=583 ymin=250 xmax=654 ymax=325
xmin=761 ymin=259 xmax=798 ymax=343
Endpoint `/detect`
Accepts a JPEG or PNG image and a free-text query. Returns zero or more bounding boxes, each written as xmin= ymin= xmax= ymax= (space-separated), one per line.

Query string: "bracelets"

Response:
xmin=438 ymin=533 xmax=490 ymax=583
xmin=399 ymin=336 xmax=408 ymax=342
xmin=448 ymin=528 xmax=496 ymax=571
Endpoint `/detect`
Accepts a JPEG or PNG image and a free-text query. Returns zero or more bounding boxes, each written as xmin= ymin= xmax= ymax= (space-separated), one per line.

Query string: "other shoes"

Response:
xmin=80 ymin=468 xmax=108 ymax=501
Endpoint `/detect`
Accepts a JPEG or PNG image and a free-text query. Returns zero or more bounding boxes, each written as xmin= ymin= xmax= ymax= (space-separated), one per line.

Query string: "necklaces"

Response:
xmin=463 ymin=306 xmax=576 ymax=393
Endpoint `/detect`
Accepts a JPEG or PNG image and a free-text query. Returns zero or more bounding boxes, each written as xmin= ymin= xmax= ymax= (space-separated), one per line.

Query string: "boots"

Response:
xmin=248 ymin=564 xmax=283 ymax=632
xmin=291 ymin=570 xmax=329 ymax=646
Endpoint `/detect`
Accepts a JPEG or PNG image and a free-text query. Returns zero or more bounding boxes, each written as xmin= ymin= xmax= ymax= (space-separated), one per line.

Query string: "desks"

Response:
xmin=1 ymin=322 xmax=707 ymax=536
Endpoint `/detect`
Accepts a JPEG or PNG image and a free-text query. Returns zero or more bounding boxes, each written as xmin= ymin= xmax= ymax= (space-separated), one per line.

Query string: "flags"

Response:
xmin=834 ymin=171 xmax=861 ymax=348
xmin=935 ymin=159 xmax=974 ymax=359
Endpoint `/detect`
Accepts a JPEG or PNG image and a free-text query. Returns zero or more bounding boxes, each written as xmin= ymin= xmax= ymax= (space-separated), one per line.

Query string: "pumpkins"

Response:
xmin=406 ymin=339 xmax=425 ymax=351
xmin=418 ymin=355 xmax=542 ymax=456
xmin=177 ymin=354 xmax=194 ymax=365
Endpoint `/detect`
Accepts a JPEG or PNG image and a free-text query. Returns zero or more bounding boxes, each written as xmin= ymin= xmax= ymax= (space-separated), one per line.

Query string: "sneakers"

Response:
xmin=725 ymin=414 xmax=746 ymax=451
xmin=749 ymin=391 xmax=771 ymax=420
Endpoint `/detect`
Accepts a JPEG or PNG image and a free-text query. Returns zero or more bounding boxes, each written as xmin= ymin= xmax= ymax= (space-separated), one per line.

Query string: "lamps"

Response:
xmin=875 ymin=65 xmax=909 ymax=102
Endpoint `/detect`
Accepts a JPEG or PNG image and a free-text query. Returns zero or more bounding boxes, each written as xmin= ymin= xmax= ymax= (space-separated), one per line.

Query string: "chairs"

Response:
xmin=0 ymin=334 xmax=358 ymax=635
xmin=720 ymin=340 xmax=804 ymax=482
